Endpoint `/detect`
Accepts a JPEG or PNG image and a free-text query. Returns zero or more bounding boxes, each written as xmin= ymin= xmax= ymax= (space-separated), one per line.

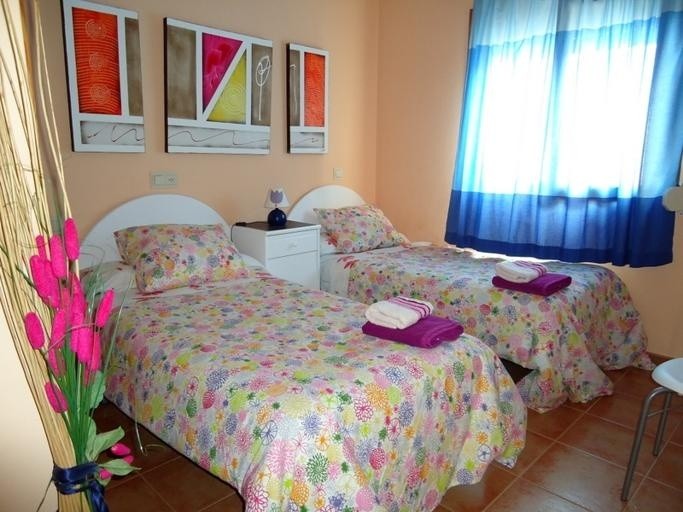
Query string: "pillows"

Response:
xmin=114 ymin=224 xmax=251 ymax=294
xmin=313 ymin=202 xmax=412 ymax=253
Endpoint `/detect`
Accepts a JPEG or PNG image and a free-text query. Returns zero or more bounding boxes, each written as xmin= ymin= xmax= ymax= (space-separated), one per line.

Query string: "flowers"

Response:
xmin=23 ymin=217 xmax=142 ymax=512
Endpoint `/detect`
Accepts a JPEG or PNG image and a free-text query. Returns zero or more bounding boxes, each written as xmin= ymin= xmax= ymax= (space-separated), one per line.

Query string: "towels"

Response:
xmin=362 ymin=318 xmax=464 ymax=349
xmin=492 ymin=273 xmax=572 ymax=296
xmin=365 ymin=295 xmax=432 ymax=330
xmin=495 ymin=259 xmax=547 ymax=283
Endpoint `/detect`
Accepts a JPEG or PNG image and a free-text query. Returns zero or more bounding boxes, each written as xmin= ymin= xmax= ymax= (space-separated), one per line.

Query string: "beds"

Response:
xmin=286 ymin=184 xmax=658 ymax=415
xmin=79 ymin=193 xmax=528 ymax=510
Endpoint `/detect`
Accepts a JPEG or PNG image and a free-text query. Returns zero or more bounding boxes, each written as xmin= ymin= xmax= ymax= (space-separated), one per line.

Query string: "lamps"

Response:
xmin=264 ymin=187 xmax=289 ymax=229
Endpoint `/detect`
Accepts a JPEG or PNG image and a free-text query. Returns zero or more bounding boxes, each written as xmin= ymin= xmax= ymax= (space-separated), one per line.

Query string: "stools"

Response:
xmin=622 ymin=359 xmax=683 ymax=500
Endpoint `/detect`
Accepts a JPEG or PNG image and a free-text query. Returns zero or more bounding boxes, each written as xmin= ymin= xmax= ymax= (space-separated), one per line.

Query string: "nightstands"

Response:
xmin=233 ymin=219 xmax=322 ymax=292
